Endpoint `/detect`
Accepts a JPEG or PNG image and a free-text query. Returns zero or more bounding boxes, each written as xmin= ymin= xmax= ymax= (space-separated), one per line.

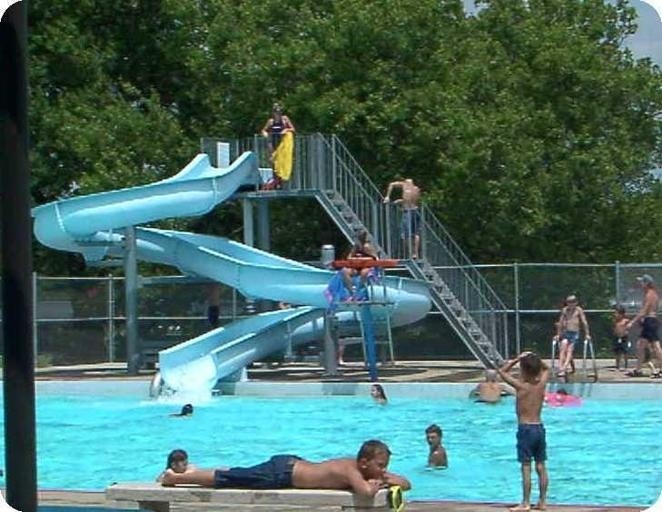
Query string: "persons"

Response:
xmin=162 ymin=439 xmax=411 ymax=499
xmin=473 ymin=368 xmax=516 ymax=404
xmin=371 ymin=384 xmax=387 ymax=403
xmin=156 ymin=448 xmax=195 ymax=483
xmin=553 ymin=274 xmax=661 ymax=395
xmin=425 ymin=422 xmax=447 ymax=467
xmin=171 ymin=403 xmax=195 ymax=418
xmin=204 ymin=282 xmax=220 ymax=327
xmin=499 ymin=351 xmax=553 ymax=512
xmin=381 ymin=172 xmax=421 ymax=260
xmin=338 ymin=335 xmax=348 ymax=367
xmin=263 ymin=103 xmax=296 ymax=188
xmin=342 ymin=229 xmax=379 ymax=301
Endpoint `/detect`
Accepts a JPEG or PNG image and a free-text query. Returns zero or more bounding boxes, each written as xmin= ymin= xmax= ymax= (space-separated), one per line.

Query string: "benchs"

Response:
xmin=104 ymin=482 xmax=402 ymax=512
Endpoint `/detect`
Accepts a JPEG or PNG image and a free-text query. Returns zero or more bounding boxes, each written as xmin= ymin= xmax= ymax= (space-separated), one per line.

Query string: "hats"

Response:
xmin=636 ymin=273 xmax=655 ymax=287
